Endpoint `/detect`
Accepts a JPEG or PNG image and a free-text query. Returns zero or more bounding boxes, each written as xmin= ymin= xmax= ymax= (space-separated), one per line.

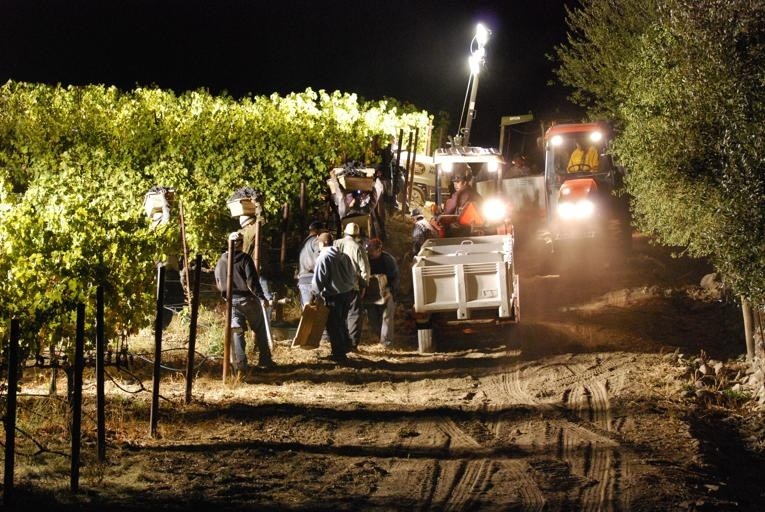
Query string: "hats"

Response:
xmin=316 ymin=233 xmax=333 ymax=241
xmin=309 ymin=221 xmax=326 ymax=231
xmin=229 ymin=232 xmax=243 ymax=247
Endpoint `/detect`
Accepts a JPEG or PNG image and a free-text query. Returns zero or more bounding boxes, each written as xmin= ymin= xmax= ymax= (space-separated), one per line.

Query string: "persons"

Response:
xmin=237 ymin=196 xmax=275 ymax=328
xmin=213 ymin=231 xmax=277 ymax=372
xmin=566 ymin=137 xmax=600 ymax=173
xmin=411 ymin=164 xmax=487 ymax=327
xmin=298 ymin=144 xmax=402 ymax=361
xmin=152 ymin=189 xmax=180 ymax=334
xmin=503 ymin=153 xmax=531 ymax=178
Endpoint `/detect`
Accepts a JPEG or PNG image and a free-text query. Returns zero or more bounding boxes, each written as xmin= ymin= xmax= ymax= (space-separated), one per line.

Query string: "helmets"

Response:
xmin=412 ymin=209 xmax=424 ymax=215
xmin=451 ymin=172 xmax=466 ymax=181
xmin=344 ymin=222 xmax=360 ymax=235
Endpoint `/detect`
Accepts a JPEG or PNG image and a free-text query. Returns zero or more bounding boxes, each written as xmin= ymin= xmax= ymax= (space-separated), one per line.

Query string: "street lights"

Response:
xmin=462 ymin=23 xmax=491 ymax=143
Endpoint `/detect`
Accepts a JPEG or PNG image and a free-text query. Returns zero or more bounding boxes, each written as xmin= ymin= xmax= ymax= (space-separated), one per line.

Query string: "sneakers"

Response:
xmin=328 ymin=354 xmax=347 ymax=361
xmin=237 ymin=361 xmax=277 ymax=374
